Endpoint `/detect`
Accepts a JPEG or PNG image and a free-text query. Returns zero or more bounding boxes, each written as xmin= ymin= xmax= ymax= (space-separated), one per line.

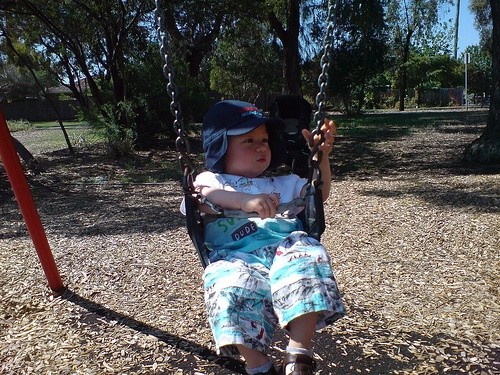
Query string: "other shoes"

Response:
xmin=281 ymin=352 xmax=316 ymax=375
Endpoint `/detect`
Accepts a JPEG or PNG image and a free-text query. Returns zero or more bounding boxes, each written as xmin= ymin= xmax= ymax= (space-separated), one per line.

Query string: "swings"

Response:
xmin=152 ymin=0 xmax=338 ymax=283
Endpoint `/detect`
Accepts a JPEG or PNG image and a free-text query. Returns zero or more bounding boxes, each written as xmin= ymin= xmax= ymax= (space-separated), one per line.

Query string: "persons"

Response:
xmin=180 ymin=100 xmax=347 ymax=375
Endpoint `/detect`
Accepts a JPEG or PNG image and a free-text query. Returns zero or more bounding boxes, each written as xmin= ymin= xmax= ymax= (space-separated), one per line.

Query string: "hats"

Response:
xmin=202 ymin=100 xmax=288 ymax=176
